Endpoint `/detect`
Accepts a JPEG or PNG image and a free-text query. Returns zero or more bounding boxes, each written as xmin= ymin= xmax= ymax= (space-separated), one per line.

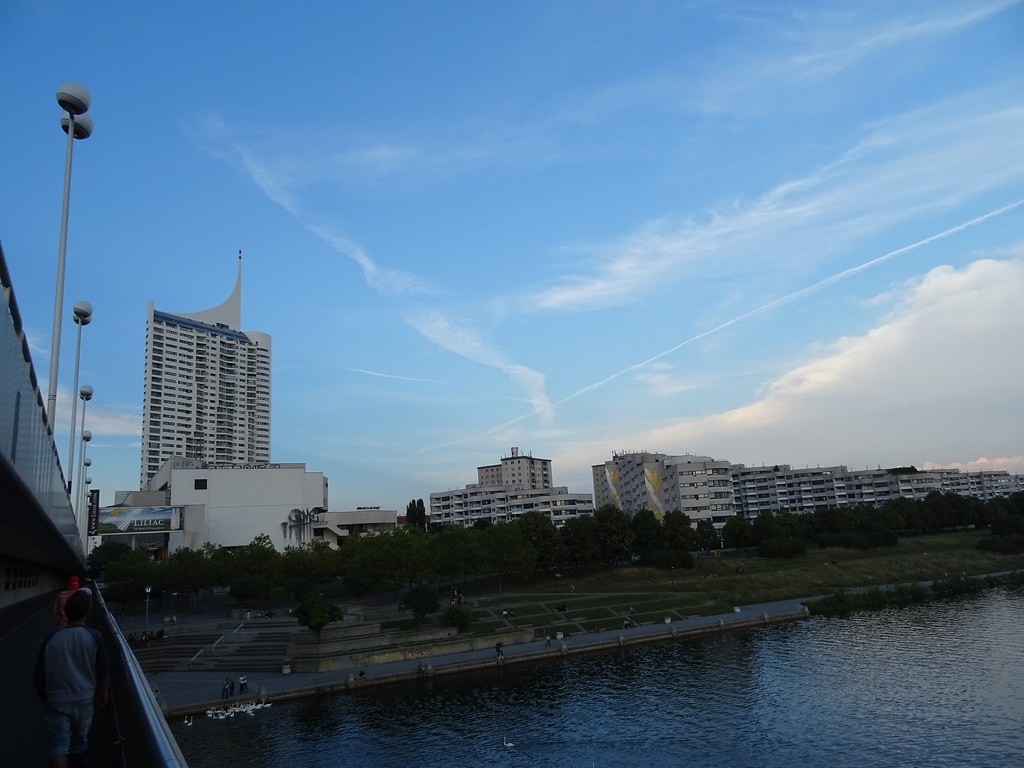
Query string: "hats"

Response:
xmin=66 ymin=576 xmax=80 ymax=590
xmin=65 ymin=588 xmax=92 ymax=616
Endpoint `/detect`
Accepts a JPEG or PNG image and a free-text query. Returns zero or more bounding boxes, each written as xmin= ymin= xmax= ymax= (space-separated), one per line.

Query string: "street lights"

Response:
xmin=143 ymin=586 xmax=153 ymax=624
xmin=64 ymin=298 xmax=93 ymax=496
xmin=78 ymin=383 xmax=94 ymax=554
xmin=43 ymin=80 xmax=94 ymax=433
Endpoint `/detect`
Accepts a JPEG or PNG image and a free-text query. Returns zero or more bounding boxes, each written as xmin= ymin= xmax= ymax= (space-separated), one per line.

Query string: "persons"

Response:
xmin=52 ymin=574 xmax=95 ymax=629
xmin=349 ymin=550 xmax=841 ymax=679
xmin=127 ymin=601 xmax=292 ymax=700
xmin=34 ymin=590 xmax=108 ymax=768
